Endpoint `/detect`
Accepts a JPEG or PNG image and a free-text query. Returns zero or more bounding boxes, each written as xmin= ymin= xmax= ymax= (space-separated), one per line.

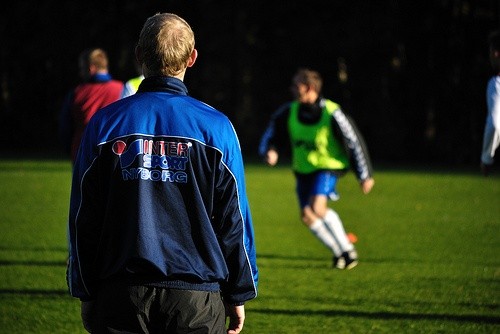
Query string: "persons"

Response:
xmin=71 ymin=14 xmax=260 ymax=334
xmin=480 ymin=31 xmax=500 ymax=185
xmin=259 ymin=68 xmax=375 ymax=268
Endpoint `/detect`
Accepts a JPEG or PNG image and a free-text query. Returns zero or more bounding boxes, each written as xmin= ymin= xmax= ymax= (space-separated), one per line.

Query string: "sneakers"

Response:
xmin=333 ymin=248 xmax=359 ymax=270
